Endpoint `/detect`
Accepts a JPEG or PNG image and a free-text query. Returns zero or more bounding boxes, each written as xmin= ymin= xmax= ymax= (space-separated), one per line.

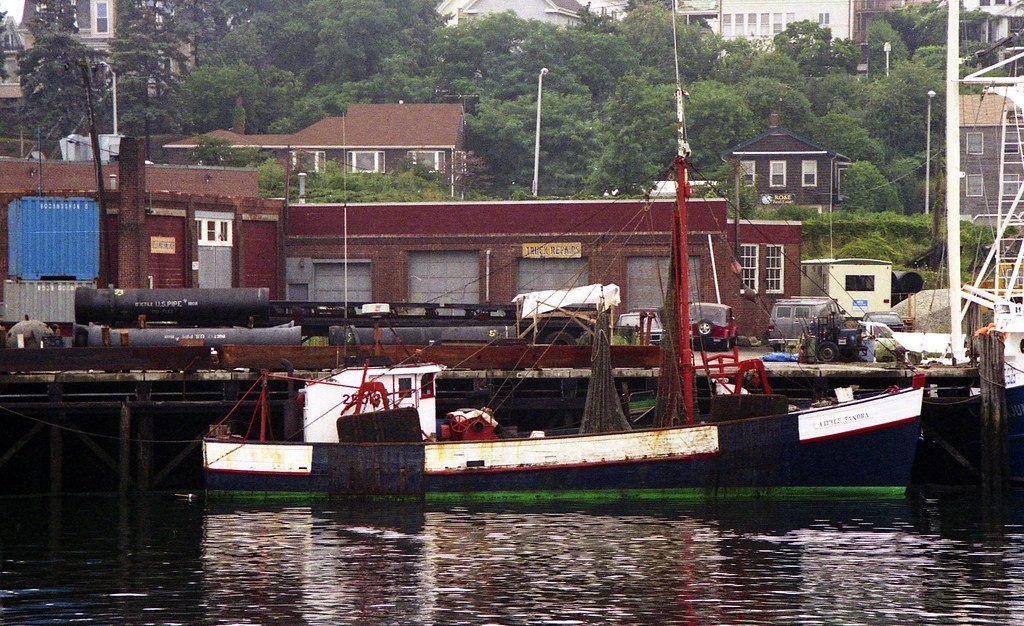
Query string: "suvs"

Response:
xmin=690 ymin=301 xmax=739 ymax=353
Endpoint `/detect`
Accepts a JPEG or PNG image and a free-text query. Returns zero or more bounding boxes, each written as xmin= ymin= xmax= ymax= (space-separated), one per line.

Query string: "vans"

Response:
xmin=768 ymin=298 xmax=838 ymax=353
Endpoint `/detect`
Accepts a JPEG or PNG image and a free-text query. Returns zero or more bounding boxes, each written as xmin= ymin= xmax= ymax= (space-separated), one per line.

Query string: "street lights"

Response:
xmin=531 ymin=68 xmax=549 ymax=198
xmin=923 ymin=90 xmax=937 ymax=213
xmin=96 ymin=61 xmax=118 ymax=138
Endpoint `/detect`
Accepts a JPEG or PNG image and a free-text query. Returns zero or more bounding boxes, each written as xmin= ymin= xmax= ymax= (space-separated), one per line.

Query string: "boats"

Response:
xmin=199 ymin=13 xmax=927 ymax=498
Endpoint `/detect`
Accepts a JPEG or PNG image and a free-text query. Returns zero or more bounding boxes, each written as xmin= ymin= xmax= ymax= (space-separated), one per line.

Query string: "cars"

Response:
xmin=862 ymin=312 xmax=905 ymax=332
xmin=616 ymin=310 xmax=665 ymax=344
